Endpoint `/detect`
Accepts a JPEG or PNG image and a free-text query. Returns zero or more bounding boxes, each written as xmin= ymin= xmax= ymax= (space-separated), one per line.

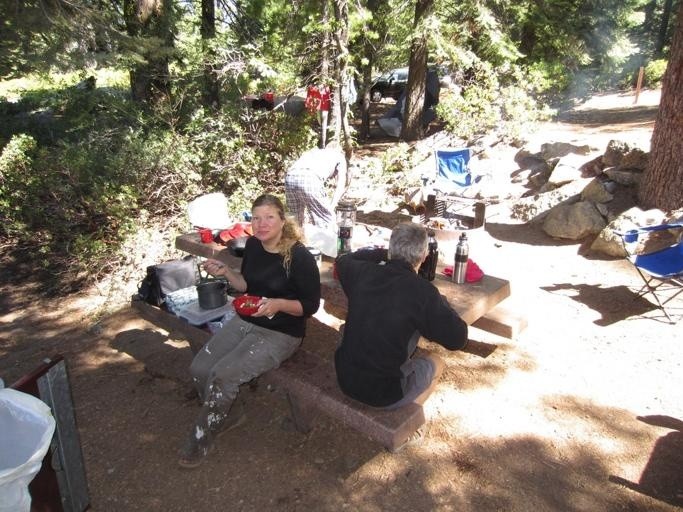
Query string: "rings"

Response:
xmin=203 ymin=264 xmax=208 ymax=271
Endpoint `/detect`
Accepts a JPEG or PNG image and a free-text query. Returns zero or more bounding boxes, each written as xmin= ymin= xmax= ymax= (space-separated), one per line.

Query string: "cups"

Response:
xmin=200 ymin=229 xmax=212 ymax=243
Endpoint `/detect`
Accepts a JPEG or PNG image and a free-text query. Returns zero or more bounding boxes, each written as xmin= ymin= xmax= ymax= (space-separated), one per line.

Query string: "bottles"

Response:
xmin=452 ymin=231 xmax=470 ymax=284
xmin=417 ymin=230 xmax=439 ymax=281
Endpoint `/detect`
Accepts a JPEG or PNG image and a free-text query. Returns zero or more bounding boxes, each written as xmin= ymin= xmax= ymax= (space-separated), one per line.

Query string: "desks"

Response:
xmin=175 ymin=220 xmax=510 ymax=326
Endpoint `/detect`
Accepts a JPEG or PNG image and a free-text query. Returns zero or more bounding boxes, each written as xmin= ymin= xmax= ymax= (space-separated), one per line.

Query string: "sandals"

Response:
xmin=178 ymin=412 xmax=247 ymax=468
xmin=389 ymin=427 xmax=426 ymax=453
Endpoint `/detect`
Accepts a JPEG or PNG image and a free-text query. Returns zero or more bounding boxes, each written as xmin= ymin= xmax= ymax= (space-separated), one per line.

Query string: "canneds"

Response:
xmin=309 ymin=249 xmax=322 ymax=269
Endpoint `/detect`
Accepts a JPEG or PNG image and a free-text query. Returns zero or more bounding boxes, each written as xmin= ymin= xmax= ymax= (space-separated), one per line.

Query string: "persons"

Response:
xmin=283 ymin=140 xmax=348 ymax=232
xmin=335 ymin=220 xmax=469 ymax=455
xmin=177 ymin=195 xmax=320 ymax=469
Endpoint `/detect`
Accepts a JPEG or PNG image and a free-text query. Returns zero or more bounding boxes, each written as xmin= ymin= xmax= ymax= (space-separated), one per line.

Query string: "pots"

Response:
xmin=197 ymin=282 xmax=228 ymax=310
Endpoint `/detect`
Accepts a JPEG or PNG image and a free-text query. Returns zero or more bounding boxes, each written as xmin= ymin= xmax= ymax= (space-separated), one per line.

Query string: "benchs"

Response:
xmin=470 ymin=305 xmax=529 ymax=340
xmin=130 ymin=281 xmax=439 ymax=452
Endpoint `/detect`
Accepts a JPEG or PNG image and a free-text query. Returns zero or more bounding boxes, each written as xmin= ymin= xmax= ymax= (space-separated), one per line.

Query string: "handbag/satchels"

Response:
xmin=138 ymin=262 xmax=194 ymax=307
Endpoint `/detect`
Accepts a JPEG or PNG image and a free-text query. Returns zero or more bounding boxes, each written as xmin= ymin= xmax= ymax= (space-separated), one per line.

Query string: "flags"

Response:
xmin=303 ymin=83 xmax=335 ymax=117
xmin=436 ymin=149 xmax=482 ymax=187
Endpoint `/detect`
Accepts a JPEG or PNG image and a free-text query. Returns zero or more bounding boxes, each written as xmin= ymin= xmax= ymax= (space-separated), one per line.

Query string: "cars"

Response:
xmin=368 ymin=66 xmax=440 ymax=109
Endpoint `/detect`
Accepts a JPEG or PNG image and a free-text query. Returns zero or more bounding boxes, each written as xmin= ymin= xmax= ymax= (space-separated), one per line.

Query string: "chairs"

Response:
xmin=424 ymin=148 xmax=487 ymax=225
xmin=613 ymin=222 xmax=683 ymax=324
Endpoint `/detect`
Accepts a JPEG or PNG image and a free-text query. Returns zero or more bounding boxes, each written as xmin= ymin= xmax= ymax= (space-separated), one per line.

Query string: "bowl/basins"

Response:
xmin=232 ymin=296 xmax=263 ymax=316
xmin=226 ymin=238 xmax=248 ymax=258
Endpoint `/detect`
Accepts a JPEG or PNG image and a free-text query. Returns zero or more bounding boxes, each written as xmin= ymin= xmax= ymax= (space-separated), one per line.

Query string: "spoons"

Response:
xmin=212 ymin=262 xmax=225 ymax=269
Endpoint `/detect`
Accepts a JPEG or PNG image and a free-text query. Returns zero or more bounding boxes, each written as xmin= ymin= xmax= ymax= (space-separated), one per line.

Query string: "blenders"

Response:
xmin=332 ymin=199 xmax=357 ymax=279
